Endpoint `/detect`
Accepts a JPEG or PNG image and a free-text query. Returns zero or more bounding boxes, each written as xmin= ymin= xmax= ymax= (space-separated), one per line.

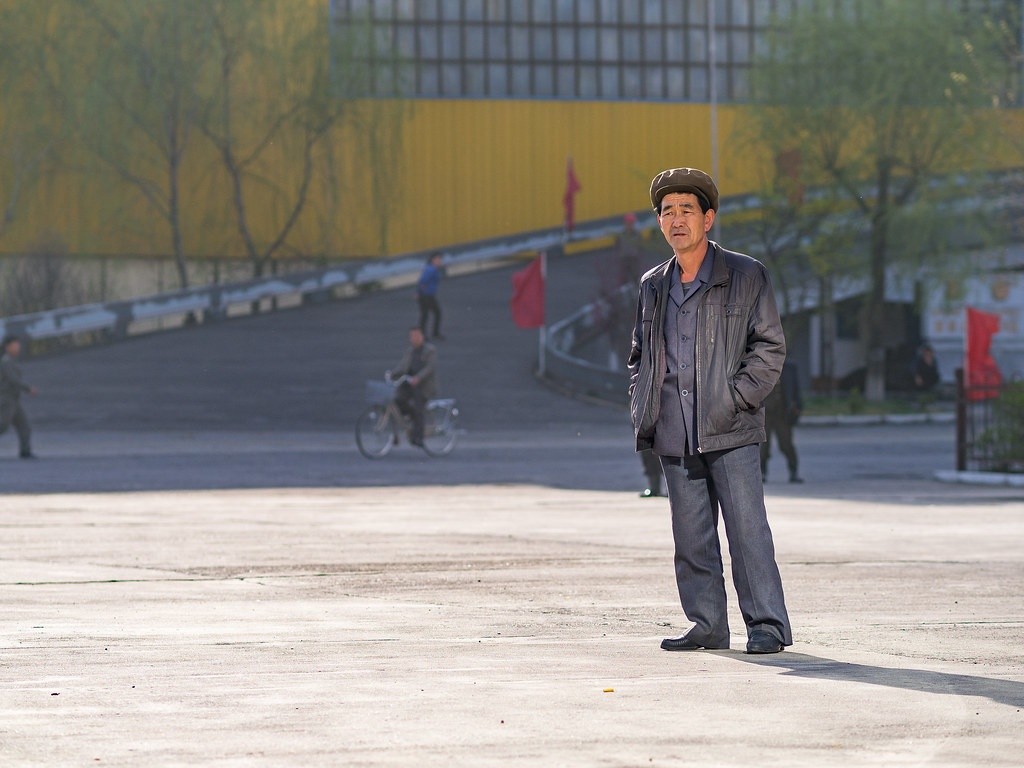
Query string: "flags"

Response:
xmin=507 ymin=254 xmax=546 ymax=327
xmin=963 ymin=304 xmax=1005 ymax=399
xmin=562 ymin=159 xmax=582 ymax=231
xmin=779 ymin=148 xmax=806 ymax=207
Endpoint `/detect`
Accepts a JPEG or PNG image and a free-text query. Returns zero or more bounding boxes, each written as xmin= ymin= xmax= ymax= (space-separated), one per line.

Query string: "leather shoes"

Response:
xmin=660 ymin=633 xmax=713 ymax=651
xmin=747 ymin=630 xmax=785 ymax=654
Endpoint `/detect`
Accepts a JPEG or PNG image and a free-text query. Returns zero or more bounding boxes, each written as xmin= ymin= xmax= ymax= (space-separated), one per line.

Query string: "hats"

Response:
xmin=650 ymin=168 xmax=720 ymax=214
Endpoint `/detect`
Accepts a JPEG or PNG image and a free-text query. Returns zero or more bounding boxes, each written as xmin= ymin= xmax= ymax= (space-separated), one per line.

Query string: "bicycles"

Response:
xmin=354 ymin=370 xmax=461 ymax=461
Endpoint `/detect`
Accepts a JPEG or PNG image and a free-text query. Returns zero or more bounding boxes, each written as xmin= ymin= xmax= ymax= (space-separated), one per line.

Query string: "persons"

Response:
xmin=416 ymin=252 xmax=445 ymax=345
xmin=629 ymin=166 xmax=795 ymax=656
xmin=761 ymin=348 xmax=803 ymax=483
xmin=639 ymin=450 xmax=669 ymax=498
xmin=914 ymin=344 xmax=940 ymax=410
xmin=0 ymin=333 xmax=39 ymax=460
xmin=388 ymin=325 xmax=438 ymax=448
